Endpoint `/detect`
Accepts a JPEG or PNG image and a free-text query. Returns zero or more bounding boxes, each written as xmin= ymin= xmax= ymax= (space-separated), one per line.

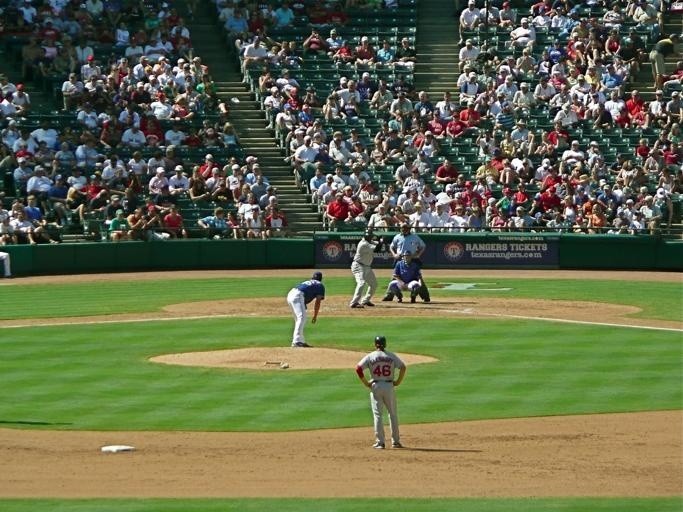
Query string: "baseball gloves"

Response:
xmin=397 ymin=281 xmax=407 ymax=291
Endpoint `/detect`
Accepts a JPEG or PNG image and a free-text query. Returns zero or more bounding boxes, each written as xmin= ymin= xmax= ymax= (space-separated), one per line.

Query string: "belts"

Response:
xmin=373 ymin=379 xmax=392 ymax=382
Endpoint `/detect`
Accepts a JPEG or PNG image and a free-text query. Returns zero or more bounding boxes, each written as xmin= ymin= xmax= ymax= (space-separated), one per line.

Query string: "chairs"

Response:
xmin=1 ymin=0 xmax=683 ymax=241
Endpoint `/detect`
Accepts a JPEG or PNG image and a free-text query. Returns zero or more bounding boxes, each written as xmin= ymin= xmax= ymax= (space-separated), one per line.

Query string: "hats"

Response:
xmin=460 ymin=0 xmax=680 ymax=218
xmin=325 ymin=1 xmax=415 ymax=223
xmin=313 ymin=272 xmax=322 ymax=279
xmin=433 ymin=110 xmax=439 ymax=115
xmin=0 ymin=7 xmax=318 ymax=223
xmin=426 ymin=130 xmax=431 ymax=136
xmin=362 ymin=229 xmax=376 ymax=237
xmin=429 ymin=200 xmax=435 ymax=204
xmin=400 ymin=224 xmax=413 ymax=228
xmin=453 ymin=112 xmax=460 ymax=117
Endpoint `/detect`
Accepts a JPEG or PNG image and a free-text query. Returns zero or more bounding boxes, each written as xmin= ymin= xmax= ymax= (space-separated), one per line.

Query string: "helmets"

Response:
xmin=375 ymin=336 xmax=386 ymax=347
xmin=402 ymin=251 xmax=412 ymax=263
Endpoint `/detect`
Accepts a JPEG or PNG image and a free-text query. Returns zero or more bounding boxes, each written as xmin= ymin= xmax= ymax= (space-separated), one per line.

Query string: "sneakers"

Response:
xmin=350 ymin=301 xmax=374 ymax=308
xmin=292 ymin=342 xmax=313 ymax=347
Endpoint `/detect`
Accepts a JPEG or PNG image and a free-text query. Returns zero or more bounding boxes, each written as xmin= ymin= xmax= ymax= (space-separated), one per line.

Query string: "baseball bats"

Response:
xmin=372 ymin=231 xmax=381 ymax=237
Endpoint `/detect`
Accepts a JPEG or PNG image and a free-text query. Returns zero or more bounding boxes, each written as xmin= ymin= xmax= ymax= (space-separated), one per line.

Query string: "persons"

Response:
xmin=0 ymin=1 xmax=682 ymax=247
xmin=346 ymin=229 xmax=377 ymax=308
xmin=354 ymin=334 xmax=407 ymax=449
xmin=378 ymin=222 xmax=430 ymax=302
xmin=286 ymin=271 xmax=325 ymax=348
xmin=0 ymin=251 xmax=16 ymax=279
xmin=388 ymin=250 xmax=419 ymax=302
